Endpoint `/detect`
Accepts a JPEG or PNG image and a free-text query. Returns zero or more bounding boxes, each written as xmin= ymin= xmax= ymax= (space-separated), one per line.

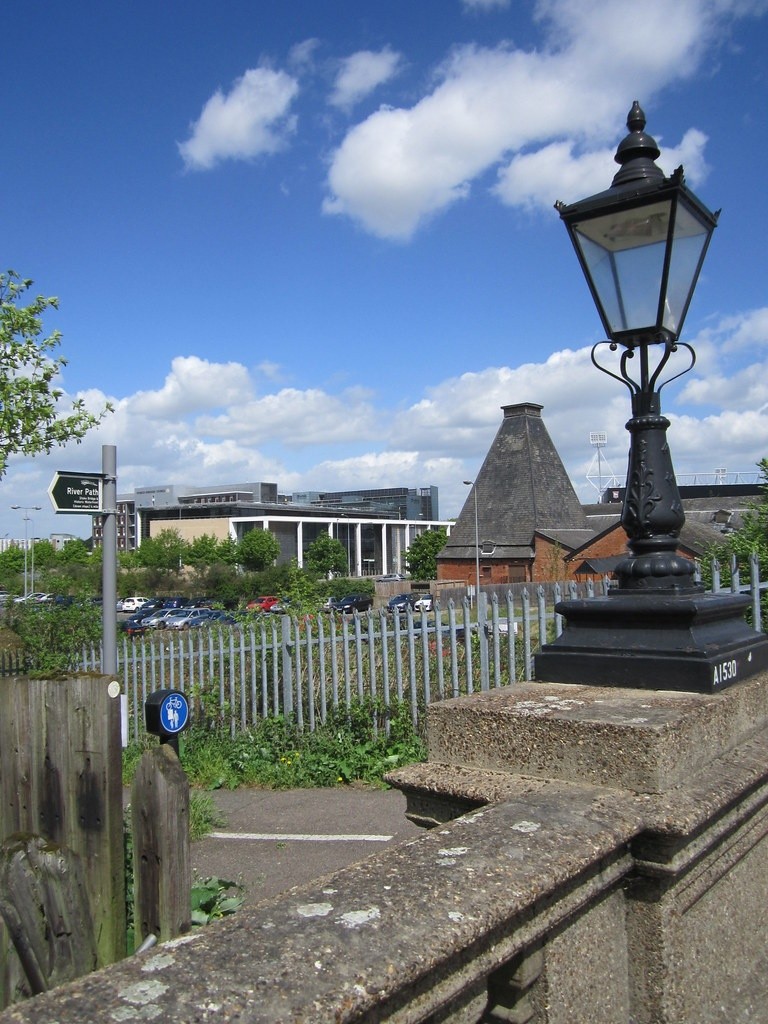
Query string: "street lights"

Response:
xmin=11 ymin=505 xmax=42 ymax=603
xmin=462 ymin=480 xmax=480 ymax=596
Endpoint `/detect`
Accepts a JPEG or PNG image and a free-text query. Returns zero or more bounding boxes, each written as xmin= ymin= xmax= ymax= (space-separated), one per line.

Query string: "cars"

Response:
xmin=88 ymin=595 xmax=293 ymax=638
xmin=319 ymin=597 xmax=341 ymax=614
xmin=333 ymin=594 xmax=374 ymax=615
xmin=414 ymin=594 xmax=433 ymax=611
xmin=386 ymin=592 xmax=421 ymax=613
xmin=0 ymin=591 xmax=75 ymax=606
xmin=376 ymin=574 xmax=406 ymax=583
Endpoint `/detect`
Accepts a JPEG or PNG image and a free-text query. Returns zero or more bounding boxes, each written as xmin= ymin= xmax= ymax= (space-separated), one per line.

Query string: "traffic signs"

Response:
xmin=48 ymin=474 xmax=104 ymax=513
